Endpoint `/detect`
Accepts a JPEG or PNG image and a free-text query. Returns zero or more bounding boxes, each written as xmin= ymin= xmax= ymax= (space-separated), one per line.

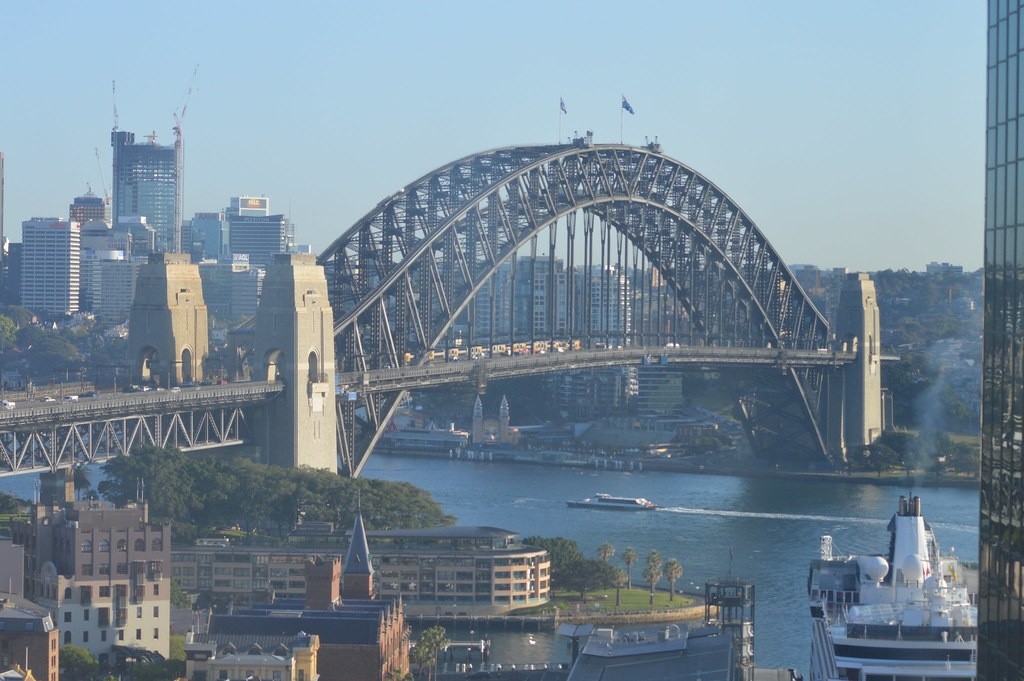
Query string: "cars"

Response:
xmin=0 ymin=378 xmax=229 ymax=409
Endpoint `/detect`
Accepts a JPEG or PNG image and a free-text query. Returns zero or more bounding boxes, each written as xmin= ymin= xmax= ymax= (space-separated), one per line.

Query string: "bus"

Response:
xmin=194 ymin=538 xmax=229 ymax=548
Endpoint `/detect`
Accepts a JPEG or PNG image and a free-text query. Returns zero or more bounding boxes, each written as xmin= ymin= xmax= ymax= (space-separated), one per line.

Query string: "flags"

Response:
xmin=622 ymin=96 xmax=634 ymax=114
xmin=560 ymin=97 xmax=567 ymax=114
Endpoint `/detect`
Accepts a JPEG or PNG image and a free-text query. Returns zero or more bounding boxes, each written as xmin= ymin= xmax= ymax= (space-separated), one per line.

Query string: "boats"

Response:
xmin=805 ymin=491 xmax=978 ymax=681
xmin=565 ymin=492 xmax=661 ymax=510
